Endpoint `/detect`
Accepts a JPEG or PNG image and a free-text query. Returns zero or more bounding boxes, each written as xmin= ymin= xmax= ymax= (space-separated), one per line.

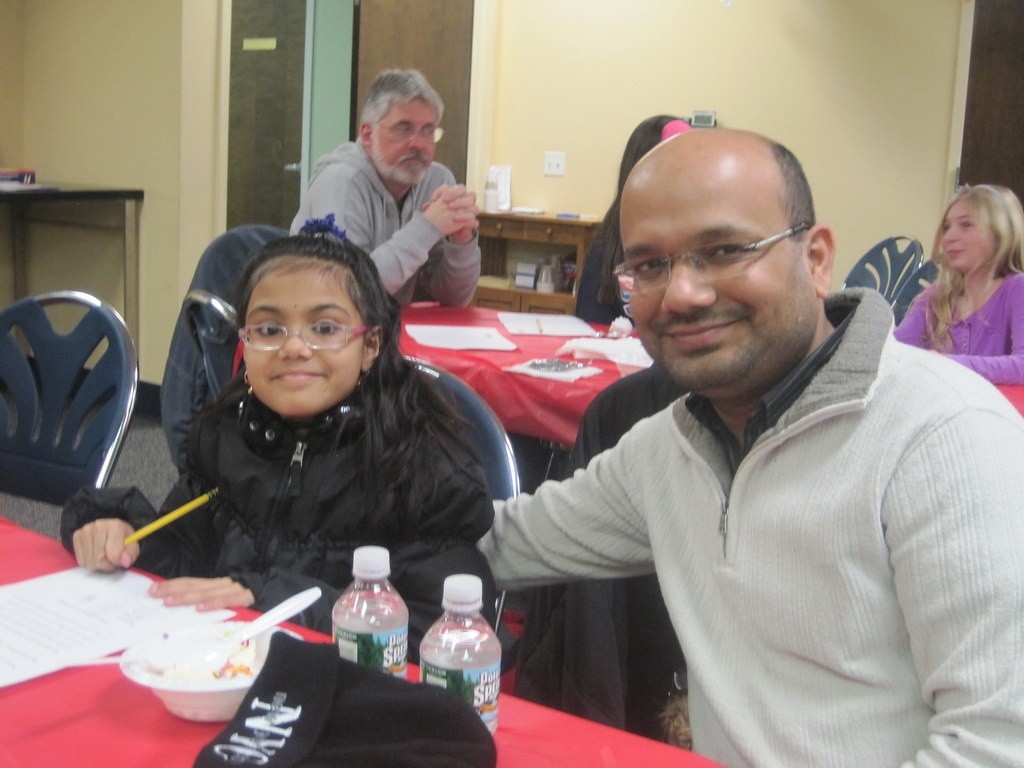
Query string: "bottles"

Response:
xmin=419 ymin=574 xmax=501 ymax=736
xmin=332 ymin=545 xmax=408 ymax=679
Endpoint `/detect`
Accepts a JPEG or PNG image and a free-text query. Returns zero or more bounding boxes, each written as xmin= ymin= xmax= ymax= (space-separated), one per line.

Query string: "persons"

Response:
xmin=481 ymin=128 xmax=1024 ymax=768
xmin=576 ymin=116 xmax=1024 ymax=391
xmin=288 ymin=69 xmax=484 ymax=311
xmin=58 ymin=229 xmax=518 ymax=684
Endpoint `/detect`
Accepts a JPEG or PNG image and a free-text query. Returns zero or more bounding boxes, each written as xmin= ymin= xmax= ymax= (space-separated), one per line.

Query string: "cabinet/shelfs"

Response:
xmin=474 ymin=209 xmax=595 ymax=316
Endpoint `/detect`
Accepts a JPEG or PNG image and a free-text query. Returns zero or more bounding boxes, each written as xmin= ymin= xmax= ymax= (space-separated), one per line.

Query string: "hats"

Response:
xmin=192 ymin=630 xmax=497 ymax=768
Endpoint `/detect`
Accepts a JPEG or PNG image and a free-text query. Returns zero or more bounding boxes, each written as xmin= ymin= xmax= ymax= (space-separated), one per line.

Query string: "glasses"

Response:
xmin=611 ymin=220 xmax=814 ymax=292
xmin=237 ymin=322 xmax=372 ymax=351
xmin=952 ymin=184 xmax=1012 ymax=225
xmin=372 ymin=122 xmax=448 ymax=144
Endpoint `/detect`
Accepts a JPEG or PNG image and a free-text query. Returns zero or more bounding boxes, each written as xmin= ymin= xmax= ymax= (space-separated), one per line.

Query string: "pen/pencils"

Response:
xmin=536 ymin=317 xmax=543 ymax=333
xmin=556 ymin=213 xmax=580 ymax=218
xmin=123 ymin=488 xmax=219 ymax=547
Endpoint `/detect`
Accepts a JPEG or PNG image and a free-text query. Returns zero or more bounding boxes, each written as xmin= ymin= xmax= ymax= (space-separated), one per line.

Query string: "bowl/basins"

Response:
xmin=120 ymin=621 xmax=302 ymax=721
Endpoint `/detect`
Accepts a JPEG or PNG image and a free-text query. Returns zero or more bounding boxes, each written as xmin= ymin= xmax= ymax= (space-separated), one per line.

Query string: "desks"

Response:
xmin=0 ymin=519 xmax=727 ymax=768
xmin=0 ymin=186 xmax=143 ymax=355
xmin=227 ymin=298 xmax=653 ymax=490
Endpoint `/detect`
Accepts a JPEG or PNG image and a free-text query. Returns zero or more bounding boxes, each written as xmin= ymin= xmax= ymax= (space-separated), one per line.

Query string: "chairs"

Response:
xmin=843 ymin=234 xmax=938 ymax=323
xmin=0 ymin=289 xmax=140 ymax=507
xmin=160 ymin=224 xmax=290 ymax=476
xmin=405 ymin=355 xmax=523 ymax=634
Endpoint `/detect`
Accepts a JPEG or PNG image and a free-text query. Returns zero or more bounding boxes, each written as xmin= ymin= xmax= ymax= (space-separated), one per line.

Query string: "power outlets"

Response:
xmin=543 ymin=150 xmax=564 ymax=175
